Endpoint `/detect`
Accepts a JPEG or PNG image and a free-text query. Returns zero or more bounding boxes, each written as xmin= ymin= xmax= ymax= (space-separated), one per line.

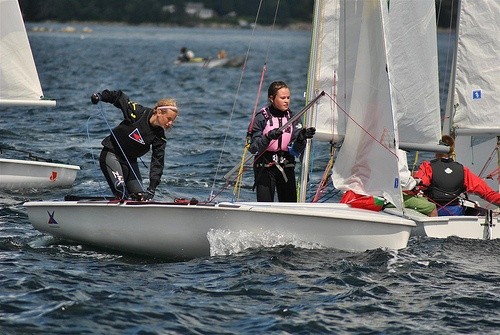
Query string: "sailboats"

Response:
xmin=0 ymin=0 xmax=81 ymax=191
xmin=385 ymin=0 xmax=500 ymax=242
xmin=22 ymin=0 xmax=417 ymax=257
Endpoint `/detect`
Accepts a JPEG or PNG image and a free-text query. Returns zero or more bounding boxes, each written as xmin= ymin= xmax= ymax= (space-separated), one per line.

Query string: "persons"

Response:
xmin=174 ymin=47 xmax=195 ymax=64
xmin=408 ymin=136 xmax=500 ymax=220
xmin=91 ymin=89 xmax=179 ymax=203
xmin=245 ymin=80 xmax=316 ymax=203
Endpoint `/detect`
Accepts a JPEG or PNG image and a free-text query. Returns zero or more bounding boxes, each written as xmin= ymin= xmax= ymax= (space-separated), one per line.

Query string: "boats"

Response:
xmin=173 ymin=55 xmax=249 ymax=70
